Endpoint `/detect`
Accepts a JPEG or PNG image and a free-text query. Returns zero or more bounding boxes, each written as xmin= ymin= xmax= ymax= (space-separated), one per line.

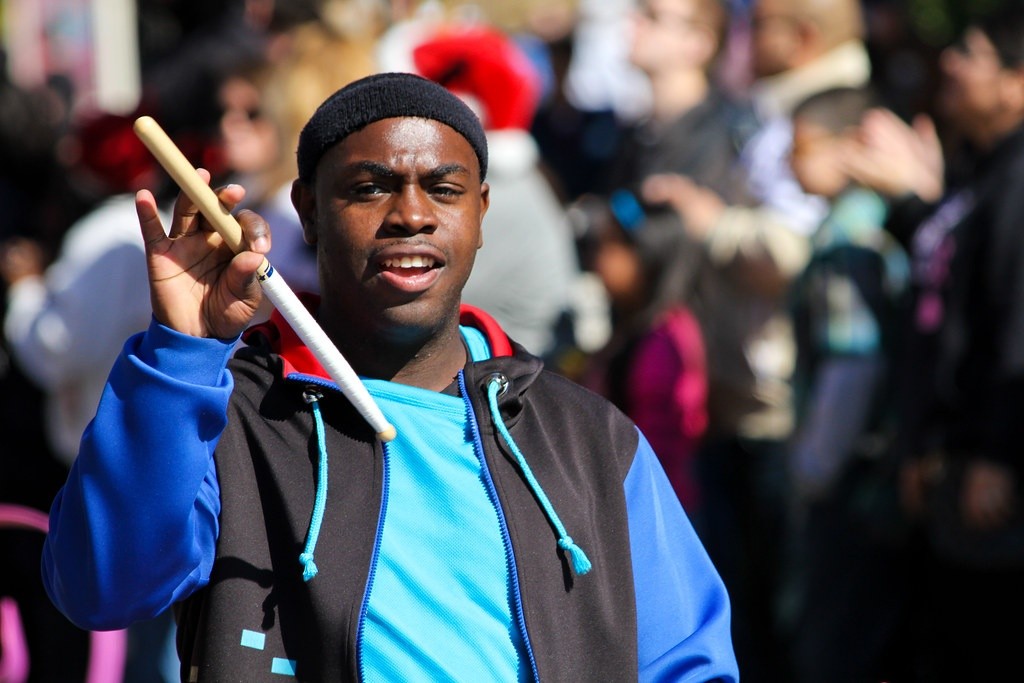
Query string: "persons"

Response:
xmin=0 ymin=0 xmax=1024 ymax=682
xmin=38 ymin=72 xmax=741 ymax=682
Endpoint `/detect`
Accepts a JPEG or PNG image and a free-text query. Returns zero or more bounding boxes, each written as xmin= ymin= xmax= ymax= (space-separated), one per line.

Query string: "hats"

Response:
xmin=412 ymin=24 xmax=542 ymax=131
xmin=297 ymin=72 xmax=488 ymax=186
